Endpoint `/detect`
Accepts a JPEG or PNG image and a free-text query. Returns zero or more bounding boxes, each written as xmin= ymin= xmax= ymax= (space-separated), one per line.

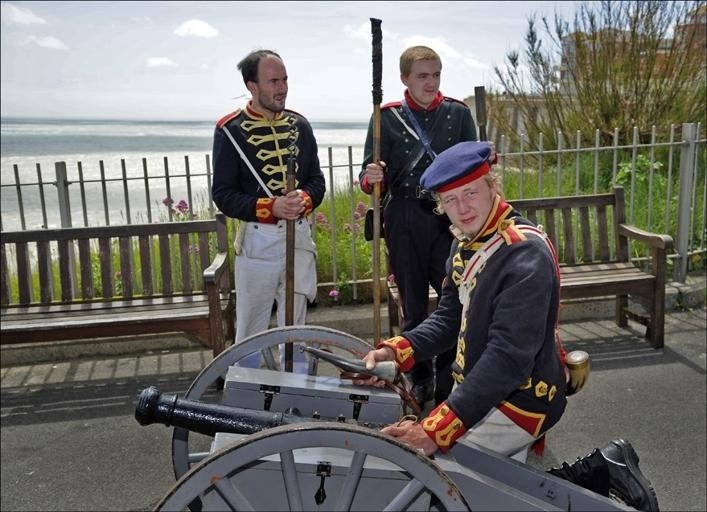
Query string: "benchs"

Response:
xmin=0 ymin=213 xmax=232 ymax=393
xmin=384 ymin=186 xmax=675 ymax=350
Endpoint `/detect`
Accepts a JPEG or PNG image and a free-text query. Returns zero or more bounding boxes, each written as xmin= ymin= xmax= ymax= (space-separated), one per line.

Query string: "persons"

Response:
xmin=358 ymin=46 xmax=498 ymax=422
xmin=211 ymin=49 xmax=326 ymax=375
xmin=352 ymin=141 xmax=662 ymax=512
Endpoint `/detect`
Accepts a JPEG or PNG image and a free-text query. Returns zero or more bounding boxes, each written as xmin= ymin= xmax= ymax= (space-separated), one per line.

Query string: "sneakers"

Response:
xmin=410 ymin=381 xmax=451 ymax=410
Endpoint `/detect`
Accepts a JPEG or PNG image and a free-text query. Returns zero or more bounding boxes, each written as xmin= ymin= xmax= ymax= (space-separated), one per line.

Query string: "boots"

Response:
xmin=544 ymin=436 xmax=660 ymax=511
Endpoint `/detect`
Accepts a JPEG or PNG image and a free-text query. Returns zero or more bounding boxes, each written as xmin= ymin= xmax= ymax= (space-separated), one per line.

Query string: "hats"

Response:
xmin=418 ymin=140 xmax=498 ymax=193
